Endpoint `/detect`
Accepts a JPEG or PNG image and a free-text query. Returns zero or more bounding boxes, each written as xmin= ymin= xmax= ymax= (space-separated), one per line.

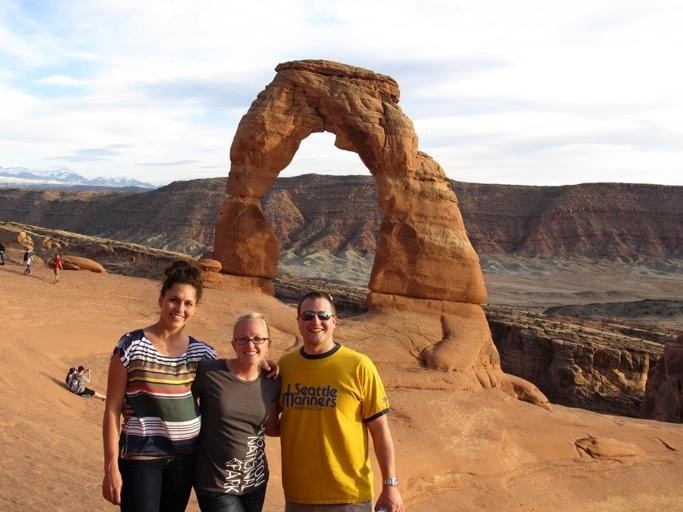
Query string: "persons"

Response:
xmin=121 ymin=402 xmax=134 ymax=422
xmin=274 ymin=292 xmax=405 ymax=512
xmin=65 ymin=365 xmax=107 ymax=401
xmin=0 ymin=244 xmax=7 ymax=264
xmin=52 ymin=253 xmax=63 ymax=282
xmin=24 ymin=249 xmax=33 ymax=274
xmin=190 ymin=313 xmax=281 ymax=512
xmin=102 ymin=262 xmax=279 ymax=512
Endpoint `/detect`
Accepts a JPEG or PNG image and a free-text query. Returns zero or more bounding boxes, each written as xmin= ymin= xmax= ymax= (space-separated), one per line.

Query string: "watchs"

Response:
xmin=381 ymin=478 xmax=399 ymax=487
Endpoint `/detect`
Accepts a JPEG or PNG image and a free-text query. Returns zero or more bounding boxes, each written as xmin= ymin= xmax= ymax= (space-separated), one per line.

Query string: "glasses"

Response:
xmin=234 ymin=337 xmax=268 ymax=344
xmin=297 ymin=311 xmax=334 ymax=321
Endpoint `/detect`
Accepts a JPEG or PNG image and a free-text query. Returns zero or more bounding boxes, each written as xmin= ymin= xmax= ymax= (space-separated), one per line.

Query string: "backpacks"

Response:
xmin=70 ymin=380 xmax=81 ymax=393
